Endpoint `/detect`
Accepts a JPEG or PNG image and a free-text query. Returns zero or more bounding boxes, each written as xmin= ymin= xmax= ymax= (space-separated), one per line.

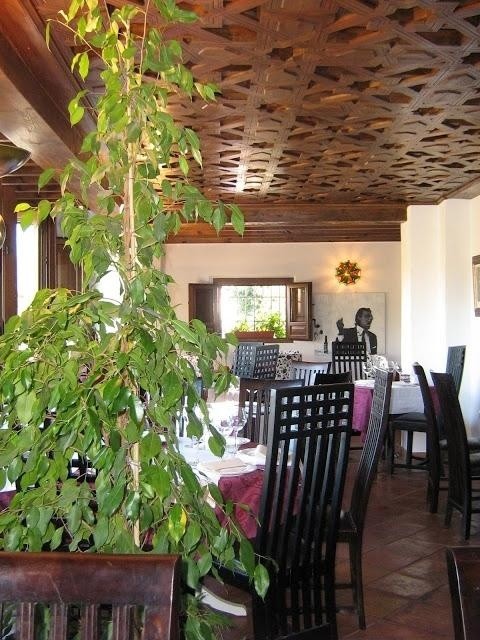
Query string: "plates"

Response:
xmin=213 ymin=463 xmax=257 ymax=477
xmin=206 ymin=436 xmax=250 ymax=453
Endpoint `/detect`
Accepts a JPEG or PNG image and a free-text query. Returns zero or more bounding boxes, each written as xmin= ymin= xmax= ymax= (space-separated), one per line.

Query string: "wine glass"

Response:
xmin=361 ymin=362 xmax=372 ymax=384
xmin=213 ymin=406 xmax=247 ymax=459
xmin=173 ymin=436 xmax=200 ymax=484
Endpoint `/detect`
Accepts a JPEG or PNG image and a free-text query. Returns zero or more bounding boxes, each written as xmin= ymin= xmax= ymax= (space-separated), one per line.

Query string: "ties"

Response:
xmin=361 ymin=330 xmax=366 ymax=352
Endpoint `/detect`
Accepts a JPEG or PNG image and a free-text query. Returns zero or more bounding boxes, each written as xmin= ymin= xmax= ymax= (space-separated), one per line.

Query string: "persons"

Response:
xmin=336 ymin=308 xmax=377 ymax=356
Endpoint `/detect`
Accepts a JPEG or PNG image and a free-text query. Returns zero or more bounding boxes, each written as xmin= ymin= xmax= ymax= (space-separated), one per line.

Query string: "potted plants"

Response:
xmin=232 ymin=312 xmax=286 ymax=341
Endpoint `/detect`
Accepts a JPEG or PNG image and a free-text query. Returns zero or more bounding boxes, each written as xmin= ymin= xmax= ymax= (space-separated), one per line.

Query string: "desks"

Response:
xmin=351 ymin=376 xmax=432 ymax=446
xmin=1 ymin=430 xmax=303 ymax=555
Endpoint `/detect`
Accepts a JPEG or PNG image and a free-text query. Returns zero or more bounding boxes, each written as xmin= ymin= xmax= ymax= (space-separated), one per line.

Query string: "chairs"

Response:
xmin=291 ymin=368 xmax=396 ymax=630
xmin=1 ymin=547 xmax=180 ymax=640
xmin=386 ymin=344 xmax=466 ymax=481
xmin=429 ymin=369 xmax=479 ymax=541
xmin=164 ymin=344 xmax=390 ymax=403
xmin=200 ymin=379 xmax=355 ymax=638
xmin=412 ymin=362 xmax=480 ymax=515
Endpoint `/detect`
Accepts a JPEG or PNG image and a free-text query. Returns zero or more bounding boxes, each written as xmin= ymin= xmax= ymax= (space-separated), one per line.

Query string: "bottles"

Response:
xmin=323 ymin=336 xmax=329 ymax=354
